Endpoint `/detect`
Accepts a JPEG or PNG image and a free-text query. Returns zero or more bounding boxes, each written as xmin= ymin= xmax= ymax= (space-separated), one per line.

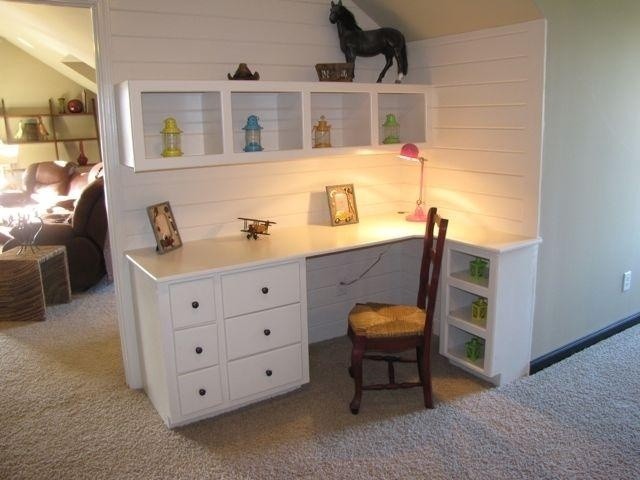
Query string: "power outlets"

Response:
xmin=621 ymin=270 xmax=633 ymax=293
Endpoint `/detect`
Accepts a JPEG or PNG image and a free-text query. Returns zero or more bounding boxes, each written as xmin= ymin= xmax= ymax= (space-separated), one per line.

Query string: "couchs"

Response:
xmin=0 ymin=160 xmax=105 ymax=293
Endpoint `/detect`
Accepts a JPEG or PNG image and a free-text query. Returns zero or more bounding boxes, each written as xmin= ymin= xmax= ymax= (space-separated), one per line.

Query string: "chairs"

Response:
xmin=346 ymin=207 xmax=448 ymax=416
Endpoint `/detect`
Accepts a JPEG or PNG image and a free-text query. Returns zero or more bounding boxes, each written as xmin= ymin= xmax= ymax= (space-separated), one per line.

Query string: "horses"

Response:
xmin=329 ymin=0 xmax=408 ymax=83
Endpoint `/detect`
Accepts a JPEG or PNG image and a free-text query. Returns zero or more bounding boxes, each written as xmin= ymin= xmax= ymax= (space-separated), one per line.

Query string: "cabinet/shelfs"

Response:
xmin=124 ymin=248 xmax=311 ymax=432
xmin=2 ymin=93 xmax=104 ymax=178
xmin=438 ymin=230 xmax=539 ymax=387
xmin=113 ymin=80 xmax=436 ymax=174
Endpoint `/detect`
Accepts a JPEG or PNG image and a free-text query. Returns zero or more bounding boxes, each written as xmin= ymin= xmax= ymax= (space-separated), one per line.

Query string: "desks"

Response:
xmin=0 ymin=245 xmax=71 ymax=322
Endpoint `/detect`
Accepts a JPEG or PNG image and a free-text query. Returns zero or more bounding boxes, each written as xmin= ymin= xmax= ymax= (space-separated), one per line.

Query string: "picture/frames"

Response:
xmin=146 ymin=201 xmax=183 ymax=254
xmin=326 ymin=183 xmax=360 ymax=227
